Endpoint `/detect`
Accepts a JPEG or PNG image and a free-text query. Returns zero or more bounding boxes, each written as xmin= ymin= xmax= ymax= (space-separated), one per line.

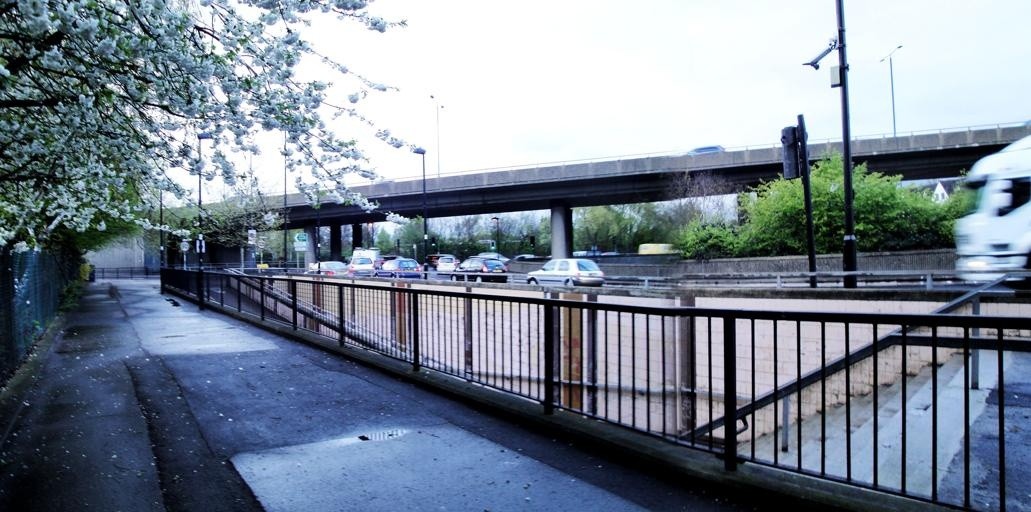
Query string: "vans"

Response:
xmin=639 ymin=243 xmax=677 ymax=254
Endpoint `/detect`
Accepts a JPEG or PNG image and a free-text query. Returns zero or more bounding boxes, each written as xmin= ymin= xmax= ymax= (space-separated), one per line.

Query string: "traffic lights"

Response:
xmin=491 ymin=242 xmax=495 ymax=250
xmin=431 ymin=238 xmax=436 ymax=247
xmin=529 ymin=236 xmax=535 ymax=247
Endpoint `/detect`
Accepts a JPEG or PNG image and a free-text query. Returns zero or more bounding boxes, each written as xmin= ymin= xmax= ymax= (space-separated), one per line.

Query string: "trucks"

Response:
xmin=951 ymin=137 xmax=1031 ymax=281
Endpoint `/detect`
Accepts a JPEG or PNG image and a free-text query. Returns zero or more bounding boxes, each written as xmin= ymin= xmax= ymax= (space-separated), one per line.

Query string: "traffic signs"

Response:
xmin=295 ymin=233 xmax=307 ymax=242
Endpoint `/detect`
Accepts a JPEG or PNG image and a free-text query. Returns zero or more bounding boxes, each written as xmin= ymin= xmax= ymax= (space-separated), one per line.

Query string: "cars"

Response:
xmin=684 ymin=146 xmax=724 ymax=154
xmin=436 ymin=257 xmax=456 ymax=271
xmin=450 ymin=258 xmax=508 ymax=282
xmin=348 ymin=247 xmax=423 ymax=279
xmin=526 ymin=259 xmax=604 ymax=286
xmin=304 ymin=262 xmax=349 ymax=275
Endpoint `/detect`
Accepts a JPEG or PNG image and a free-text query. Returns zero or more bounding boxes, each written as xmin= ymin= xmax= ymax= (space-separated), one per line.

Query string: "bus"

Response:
xmin=425 ymin=254 xmax=453 ymax=265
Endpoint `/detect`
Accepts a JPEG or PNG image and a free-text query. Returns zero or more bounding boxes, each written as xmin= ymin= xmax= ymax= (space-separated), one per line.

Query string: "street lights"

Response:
xmin=430 ymin=95 xmax=445 ymax=174
xmin=198 ymin=133 xmax=213 ymax=271
xmin=880 ymin=46 xmax=905 ymax=135
xmin=413 ymin=147 xmax=428 ymax=254
